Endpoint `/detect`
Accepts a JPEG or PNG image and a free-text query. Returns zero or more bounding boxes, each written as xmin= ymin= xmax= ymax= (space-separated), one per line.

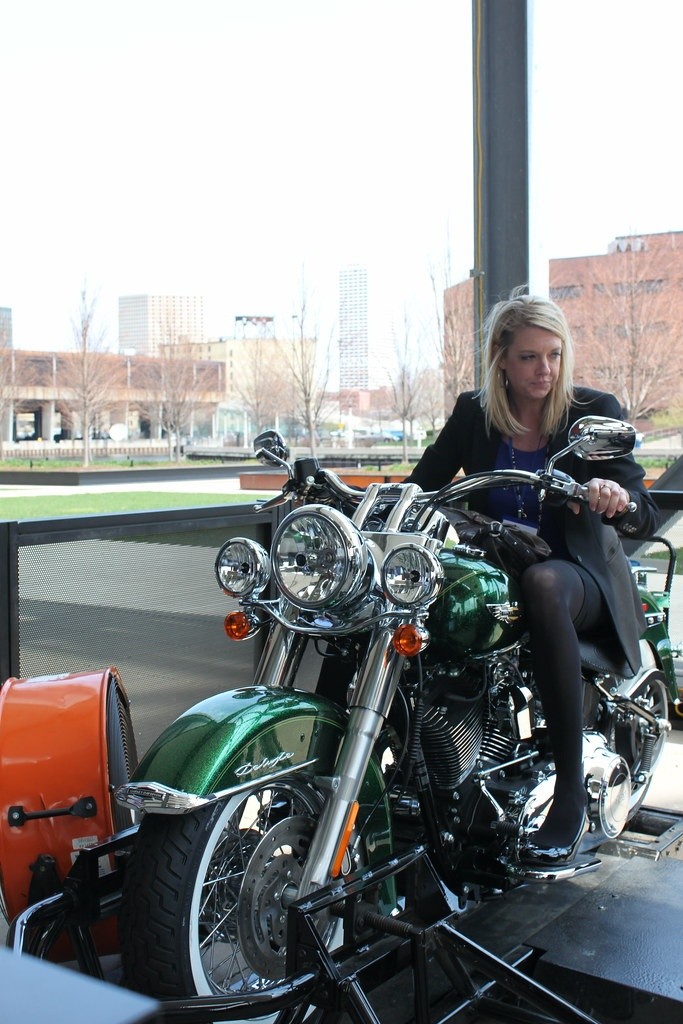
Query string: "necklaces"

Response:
xmin=511 ymin=448 xmax=528 ymax=520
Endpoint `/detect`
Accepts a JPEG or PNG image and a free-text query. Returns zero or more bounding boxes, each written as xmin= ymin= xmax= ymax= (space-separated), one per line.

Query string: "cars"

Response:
xmin=376 ymin=429 xmax=404 ymax=441
xmin=359 ymin=431 xmax=399 ymax=444
xmin=612 ymin=453 xmax=683 ymax=718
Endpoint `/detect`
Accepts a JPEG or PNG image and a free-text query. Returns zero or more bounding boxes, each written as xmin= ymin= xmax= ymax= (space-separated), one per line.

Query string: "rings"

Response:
xmin=600 ymin=484 xmax=611 ymax=491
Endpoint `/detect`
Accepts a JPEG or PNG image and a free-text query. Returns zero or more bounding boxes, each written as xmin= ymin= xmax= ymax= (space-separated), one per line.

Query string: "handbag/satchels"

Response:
xmin=437 ymin=504 xmax=552 ymax=582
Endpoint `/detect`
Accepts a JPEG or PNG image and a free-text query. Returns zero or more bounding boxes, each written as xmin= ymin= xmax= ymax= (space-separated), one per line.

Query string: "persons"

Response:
xmin=397 ymin=284 xmax=662 ymax=867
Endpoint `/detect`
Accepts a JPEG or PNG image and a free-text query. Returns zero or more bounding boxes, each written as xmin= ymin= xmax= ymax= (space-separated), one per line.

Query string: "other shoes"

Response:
xmin=519 ymin=806 xmax=590 ymax=866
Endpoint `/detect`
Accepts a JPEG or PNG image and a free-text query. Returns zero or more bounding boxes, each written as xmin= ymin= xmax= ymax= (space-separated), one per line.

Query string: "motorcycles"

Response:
xmin=113 ymin=415 xmax=683 ymax=1024
